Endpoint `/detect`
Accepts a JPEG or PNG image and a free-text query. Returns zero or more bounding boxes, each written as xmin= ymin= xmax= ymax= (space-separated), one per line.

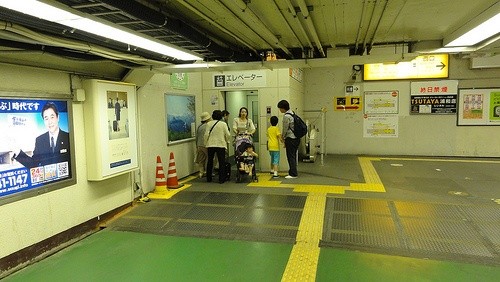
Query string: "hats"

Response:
xmin=200 ymin=112 xmax=211 ymax=121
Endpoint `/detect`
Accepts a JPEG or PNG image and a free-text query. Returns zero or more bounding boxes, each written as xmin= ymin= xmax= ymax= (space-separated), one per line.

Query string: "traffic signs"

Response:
xmin=371 ymin=61 xmax=446 ymax=75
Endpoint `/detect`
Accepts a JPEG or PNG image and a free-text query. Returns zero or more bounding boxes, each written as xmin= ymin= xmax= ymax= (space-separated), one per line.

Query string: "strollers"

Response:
xmin=235 ymin=134 xmax=258 ymax=183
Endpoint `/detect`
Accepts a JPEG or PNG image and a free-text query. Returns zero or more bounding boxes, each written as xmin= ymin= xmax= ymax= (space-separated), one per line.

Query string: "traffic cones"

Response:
xmin=168 ymin=152 xmax=184 ymax=188
xmin=156 ymin=156 xmax=167 ymax=186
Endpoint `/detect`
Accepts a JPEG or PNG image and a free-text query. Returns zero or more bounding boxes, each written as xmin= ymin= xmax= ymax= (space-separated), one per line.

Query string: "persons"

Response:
xmin=9 ymin=102 xmax=69 ymax=168
xmin=196 ymin=107 xmax=259 ymax=184
xmin=108 ymin=97 xmax=127 ymax=123
xmin=267 ymin=116 xmax=286 ymax=179
xmin=277 ymin=100 xmax=300 ymax=179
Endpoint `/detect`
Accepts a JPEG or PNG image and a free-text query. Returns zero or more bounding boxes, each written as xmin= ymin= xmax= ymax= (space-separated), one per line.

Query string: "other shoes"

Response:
xmin=285 ymin=175 xmax=297 ymax=178
xmin=219 ymin=179 xmax=225 ymax=184
xmin=270 ymin=171 xmax=274 ymax=174
xmin=273 ymin=175 xmax=280 ymax=178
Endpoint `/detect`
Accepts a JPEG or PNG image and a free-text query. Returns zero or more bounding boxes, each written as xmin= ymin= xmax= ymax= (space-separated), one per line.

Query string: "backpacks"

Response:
xmin=285 ymin=111 xmax=307 ymax=138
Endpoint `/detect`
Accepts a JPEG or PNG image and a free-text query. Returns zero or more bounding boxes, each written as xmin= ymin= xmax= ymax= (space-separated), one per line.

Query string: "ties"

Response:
xmin=51 ymin=137 xmax=54 ymax=153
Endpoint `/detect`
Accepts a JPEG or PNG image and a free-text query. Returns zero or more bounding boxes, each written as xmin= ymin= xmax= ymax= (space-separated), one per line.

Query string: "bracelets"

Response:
xmin=251 ymin=152 xmax=254 ymax=155
